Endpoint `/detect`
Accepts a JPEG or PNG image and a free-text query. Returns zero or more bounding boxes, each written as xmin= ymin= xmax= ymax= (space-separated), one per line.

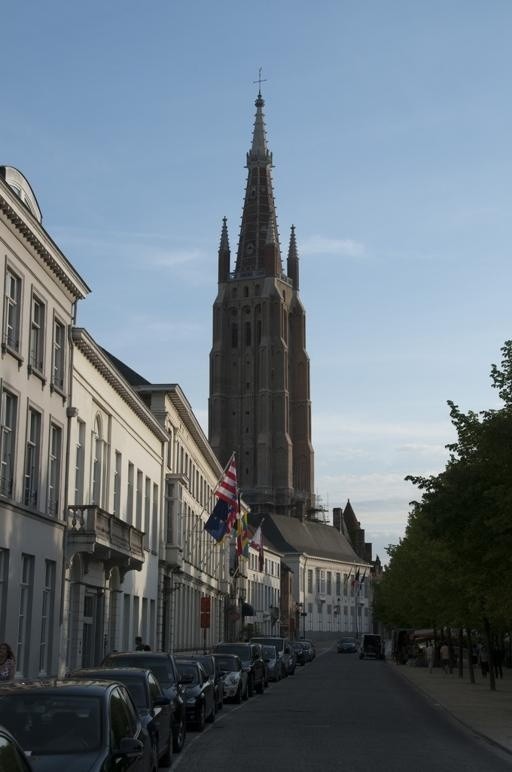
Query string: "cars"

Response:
xmin=67 ymin=632 xmax=319 ymax=772
xmin=335 ymin=636 xmax=358 ymax=654
xmin=0 ymin=720 xmax=36 ymax=772
xmin=0 ymin=674 xmax=160 ymax=772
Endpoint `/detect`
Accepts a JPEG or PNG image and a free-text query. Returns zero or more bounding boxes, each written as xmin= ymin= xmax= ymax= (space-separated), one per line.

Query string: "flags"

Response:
xmin=250 ymin=523 xmax=265 ymax=572
xmin=236 ymin=510 xmax=252 ymax=561
xmin=358 ymin=570 xmax=365 ymax=597
xmin=204 ymin=493 xmax=228 ymax=545
xmin=214 ymin=453 xmax=237 ymax=533
xmin=351 ymin=569 xmax=359 ymax=589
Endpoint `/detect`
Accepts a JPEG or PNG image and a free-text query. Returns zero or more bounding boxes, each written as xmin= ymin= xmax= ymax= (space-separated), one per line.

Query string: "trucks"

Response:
xmin=357 ymin=634 xmax=387 ymax=660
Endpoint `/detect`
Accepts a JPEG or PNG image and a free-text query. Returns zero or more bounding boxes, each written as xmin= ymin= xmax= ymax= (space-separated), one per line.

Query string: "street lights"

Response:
xmin=300 ymin=612 xmax=308 ymax=640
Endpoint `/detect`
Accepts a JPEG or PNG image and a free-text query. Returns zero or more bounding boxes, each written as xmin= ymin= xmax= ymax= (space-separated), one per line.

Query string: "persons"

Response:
xmin=491 ymin=644 xmax=503 ymax=679
xmin=477 ymin=645 xmax=489 ymax=680
xmin=0 ymin=642 xmax=17 ymax=684
xmin=135 ymin=634 xmax=152 ymax=651
xmin=425 ymin=643 xmax=436 ymax=674
xmin=438 ymin=641 xmax=451 ymax=675
xmin=470 ymin=643 xmax=482 ymax=681
